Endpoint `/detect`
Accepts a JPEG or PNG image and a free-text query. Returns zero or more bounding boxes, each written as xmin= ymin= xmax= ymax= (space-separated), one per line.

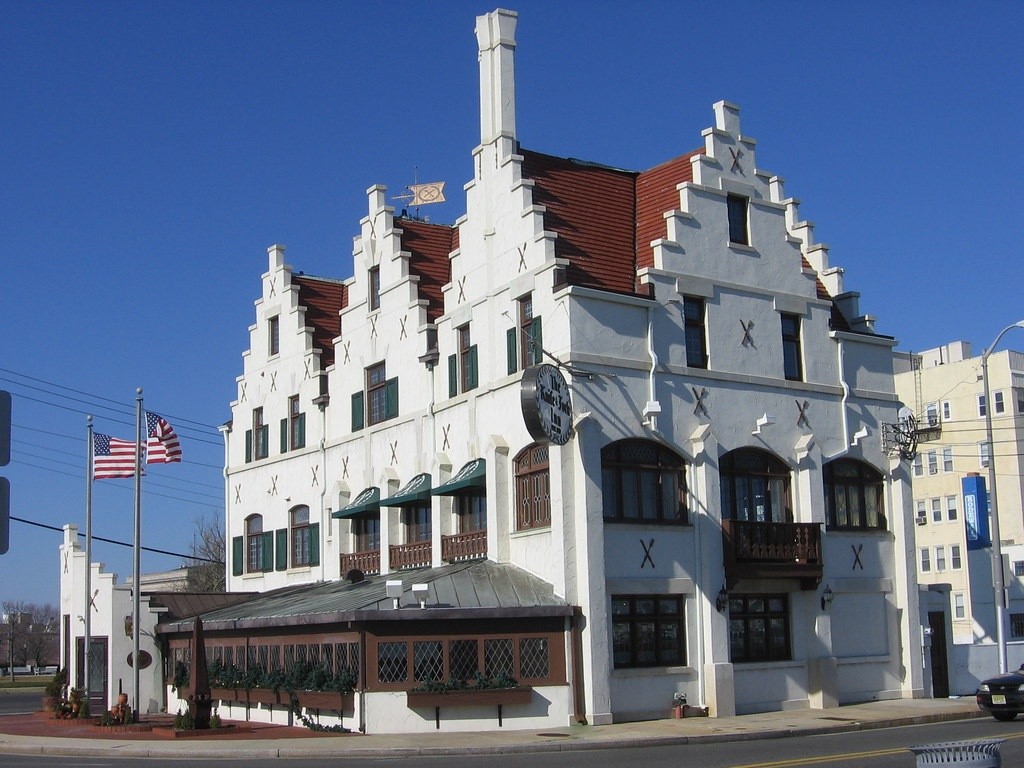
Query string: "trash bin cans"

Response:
xmin=909 ymin=739 xmax=1008 ymax=768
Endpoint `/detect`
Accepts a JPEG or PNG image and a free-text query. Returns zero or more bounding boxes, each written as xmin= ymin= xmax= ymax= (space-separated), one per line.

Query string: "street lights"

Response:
xmin=981 ymin=316 xmax=1024 ymax=676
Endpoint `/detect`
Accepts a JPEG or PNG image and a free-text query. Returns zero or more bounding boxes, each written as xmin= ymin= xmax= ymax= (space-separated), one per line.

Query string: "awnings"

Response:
xmin=430 ymin=458 xmax=486 ymax=496
xmin=332 ymin=489 xmax=380 ymax=519
xmin=379 ymin=473 xmax=431 ymax=506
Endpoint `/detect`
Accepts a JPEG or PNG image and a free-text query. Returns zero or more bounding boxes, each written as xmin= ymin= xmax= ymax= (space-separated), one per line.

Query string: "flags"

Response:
xmin=93 ymin=432 xmax=146 ymax=479
xmin=145 ymin=411 xmax=182 ymax=464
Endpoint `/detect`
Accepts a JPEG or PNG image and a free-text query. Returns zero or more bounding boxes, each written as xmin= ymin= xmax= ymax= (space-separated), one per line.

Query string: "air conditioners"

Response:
xmin=929 ymin=419 xmax=937 ymax=425
xmin=915 ymin=517 xmax=926 ymax=524
xmin=930 ymin=468 xmax=937 ymax=474
xmin=981 ymin=460 xmax=989 ymax=467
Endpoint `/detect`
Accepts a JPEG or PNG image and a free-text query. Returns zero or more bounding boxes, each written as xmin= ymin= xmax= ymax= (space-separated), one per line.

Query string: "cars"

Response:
xmin=974 ymin=662 xmax=1024 ymax=722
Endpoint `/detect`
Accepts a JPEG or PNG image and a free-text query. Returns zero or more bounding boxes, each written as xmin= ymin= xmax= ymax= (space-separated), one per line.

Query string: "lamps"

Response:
xmin=77 ymin=613 xmax=85 ymax=623
xmin=716 ymin=584 xmax=729 ymax=613
xmin=412 ymin=584 xmax=430 ymax=610
xmin=41 ymin=667 xmax=68 ymax=712
xmin=386 ymin=580 xmax=403 ymax=610
xmin=821 ymin=584 xmax=833 ymax=610
